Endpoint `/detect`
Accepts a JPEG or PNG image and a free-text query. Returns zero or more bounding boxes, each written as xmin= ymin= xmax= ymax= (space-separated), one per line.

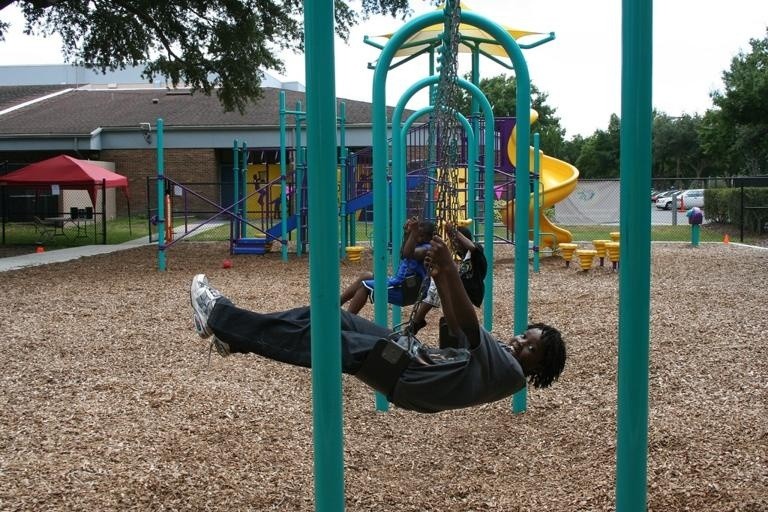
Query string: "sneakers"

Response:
xmin=188 ymin=273 xmax=232 ymax=359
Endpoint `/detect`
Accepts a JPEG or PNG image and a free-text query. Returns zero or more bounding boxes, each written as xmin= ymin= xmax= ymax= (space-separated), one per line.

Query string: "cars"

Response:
xmin=650 ymin=188 xmax=704 ymax=210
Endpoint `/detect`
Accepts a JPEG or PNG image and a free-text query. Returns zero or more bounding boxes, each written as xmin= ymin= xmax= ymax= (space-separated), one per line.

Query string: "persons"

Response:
xmin=286 ymin=182 xmax=293 ymax=207
xmin=270 ymin=194 xmax=281 ymax=219
xmin=252 ymin=174 xmax=266 ymax=205
xmin=403 ymin=222 xmax=488 ymax=335
xmin=340 ymin=215 xmax=434 ymax=315
xmin=189 ymin=233 xmax=566 ymax=414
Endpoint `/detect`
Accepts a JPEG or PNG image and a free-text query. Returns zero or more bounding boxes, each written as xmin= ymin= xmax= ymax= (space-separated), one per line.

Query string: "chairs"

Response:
xmin=34 ymin=215 xmax=55 ymax=239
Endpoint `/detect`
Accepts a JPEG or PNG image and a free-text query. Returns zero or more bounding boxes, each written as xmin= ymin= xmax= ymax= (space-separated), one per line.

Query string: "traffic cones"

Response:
xmin=724 ymin=234 xmax=728 ymax=244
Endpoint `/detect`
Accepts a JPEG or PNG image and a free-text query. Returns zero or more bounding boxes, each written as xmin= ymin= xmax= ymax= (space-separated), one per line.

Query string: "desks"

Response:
xmin=45 ymin=217 xmax=92 ymax=240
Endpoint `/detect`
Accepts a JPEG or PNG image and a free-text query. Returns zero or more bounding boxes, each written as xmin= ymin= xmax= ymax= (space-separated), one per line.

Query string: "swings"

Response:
xmin=355 ymin=0 xmax=463 ymax=401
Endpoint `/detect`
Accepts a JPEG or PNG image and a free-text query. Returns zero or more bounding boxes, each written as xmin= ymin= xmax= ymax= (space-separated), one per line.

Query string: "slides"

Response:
xmin=500 ymin=109 xmax=580 ymax=247
